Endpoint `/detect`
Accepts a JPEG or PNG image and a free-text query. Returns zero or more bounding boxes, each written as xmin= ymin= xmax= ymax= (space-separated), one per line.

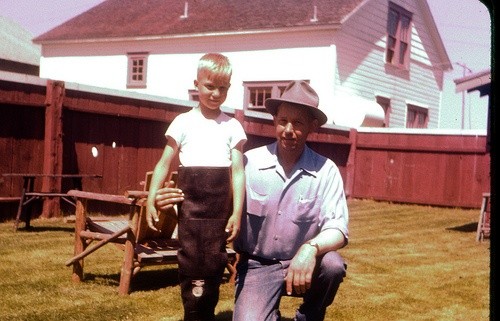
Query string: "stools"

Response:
xmin=476 ymin=192 xmax=491 ymax=244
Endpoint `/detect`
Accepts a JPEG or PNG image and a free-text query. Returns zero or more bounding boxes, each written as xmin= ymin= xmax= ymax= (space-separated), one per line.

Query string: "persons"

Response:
xmin=155 ymin=81 xmax=349 ymax=321
xmin=145 ymin=52 xmax=248 ymax=321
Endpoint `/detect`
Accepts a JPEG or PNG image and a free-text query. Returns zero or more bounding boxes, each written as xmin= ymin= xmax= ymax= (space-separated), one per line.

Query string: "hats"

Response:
xmin=264 ymin=80 xmax=327 ymax=127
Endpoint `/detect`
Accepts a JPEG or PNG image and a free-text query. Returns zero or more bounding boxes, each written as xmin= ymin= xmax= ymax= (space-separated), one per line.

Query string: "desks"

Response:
xmin=3 ymin=172 xmax=104 ymax=233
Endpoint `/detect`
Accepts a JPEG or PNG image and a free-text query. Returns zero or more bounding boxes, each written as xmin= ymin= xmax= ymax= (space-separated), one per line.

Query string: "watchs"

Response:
xmin=303 ymin=241 xmax=320 ymax=256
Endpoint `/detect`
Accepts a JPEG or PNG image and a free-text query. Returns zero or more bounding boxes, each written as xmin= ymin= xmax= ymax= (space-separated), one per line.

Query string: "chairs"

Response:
xmin=67 ymin=169 xmax=241 ymax=296
